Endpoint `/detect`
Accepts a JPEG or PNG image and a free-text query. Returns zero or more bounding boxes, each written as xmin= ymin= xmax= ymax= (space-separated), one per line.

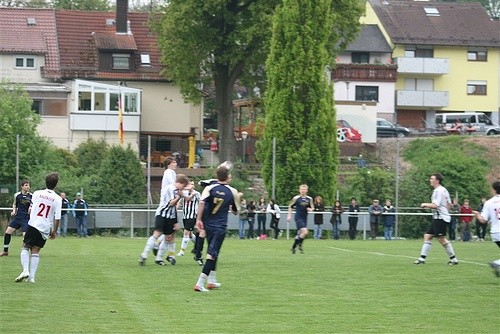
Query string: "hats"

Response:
xmin=75 ymin=192 xmax=81 ymax=196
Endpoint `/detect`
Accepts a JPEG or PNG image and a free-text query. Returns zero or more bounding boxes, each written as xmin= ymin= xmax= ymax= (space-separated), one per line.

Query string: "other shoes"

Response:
xmin=166 ymin=256 xmax=176 ymax=265
xmin=490 ymin=258 xmax=500 ymax=278
xmin=176 ymin=249 xmax=185 ymax=257
xmin=206 ymin=282 xmax=221 ymax=289
xmin=0 ymin=251 xmax=8 ymax=256
xmin=289 ymin=246 xmax=296 ymax=255
xmin=193 ymin=283 xmax=209 ymax=292
xmin=138 ymin=255 xmax=147 ymax=263
xmin=152 ymin=247 xmax=158 ymax=256
xmin=447 ymin=257 xmax=458 ymax=265
xmin=298 ymin=246 xmax=304 ymax=254
xmin=14 ymin=270 xmax=30 ymax=282
xmin=411 ymin=257 xmax=425 ymax=265
xmin=475 ymin=237 xmax=484 ymax=242
xmin=25 ymin=277 xmax=34 ymax=283
xmin=154 ymin=259 xmax=165 ymax=266
xmin=193 ymin=254 xmax=204 ymax=266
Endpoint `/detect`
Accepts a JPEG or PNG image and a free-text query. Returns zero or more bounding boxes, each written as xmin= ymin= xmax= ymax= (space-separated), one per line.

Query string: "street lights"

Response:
xmin=241 ymin=131 xmax=248 ymax=168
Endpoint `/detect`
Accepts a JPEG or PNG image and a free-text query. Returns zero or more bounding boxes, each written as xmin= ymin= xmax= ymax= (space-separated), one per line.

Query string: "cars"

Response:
xmin=377 ymin=118 xmax=409 ymax=138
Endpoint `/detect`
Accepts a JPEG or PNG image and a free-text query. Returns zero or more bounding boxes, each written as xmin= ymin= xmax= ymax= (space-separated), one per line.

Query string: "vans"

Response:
xmin=435 ymin=113 xmax=500 ymax=135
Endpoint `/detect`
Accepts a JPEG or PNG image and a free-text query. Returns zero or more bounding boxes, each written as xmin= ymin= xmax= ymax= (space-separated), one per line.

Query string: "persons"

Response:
xmin=138 ymin=157 xmax=395 ymax=292
xmin=15 ymin=173 xmax=62 ymax=283
xmin=414 ymin=174 xmax=500 ymax=279
xmin=72 ymin=193 xmax=90 ymax=238
xmin=0 ymin=180 xmax=33 ymax=257
xmin=57 ymin=192 xmax=70 ymax=237
